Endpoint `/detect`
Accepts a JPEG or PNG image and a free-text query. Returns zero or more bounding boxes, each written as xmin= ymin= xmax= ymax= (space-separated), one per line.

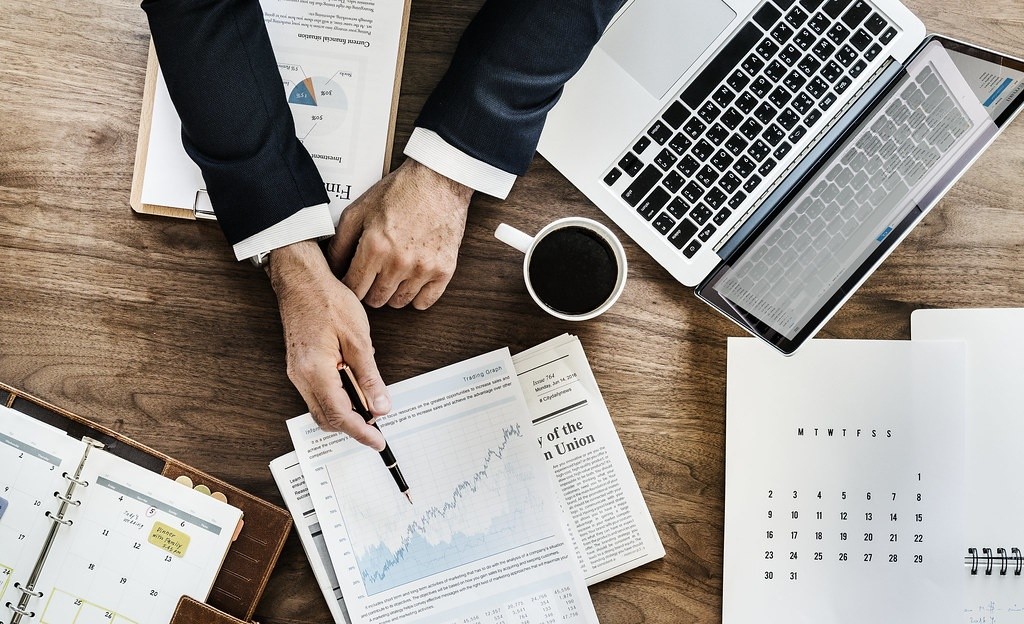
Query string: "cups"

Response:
xmin=494 ymin=216 xmax=627 ymax=321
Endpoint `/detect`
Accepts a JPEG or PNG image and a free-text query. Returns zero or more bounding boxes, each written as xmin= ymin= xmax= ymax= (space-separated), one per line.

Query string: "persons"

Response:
xmin=141 ymin=0 xmax=624 ymax=452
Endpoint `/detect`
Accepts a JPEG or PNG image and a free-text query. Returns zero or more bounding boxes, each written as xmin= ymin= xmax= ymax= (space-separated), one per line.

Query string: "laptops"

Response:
xmin=537 ymin=0 xmax=1024 ymax=355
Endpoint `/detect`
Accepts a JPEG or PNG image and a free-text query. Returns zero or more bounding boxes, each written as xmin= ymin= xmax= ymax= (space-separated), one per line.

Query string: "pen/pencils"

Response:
xmin=337 ymin=363 xmax=413 ymax=505
